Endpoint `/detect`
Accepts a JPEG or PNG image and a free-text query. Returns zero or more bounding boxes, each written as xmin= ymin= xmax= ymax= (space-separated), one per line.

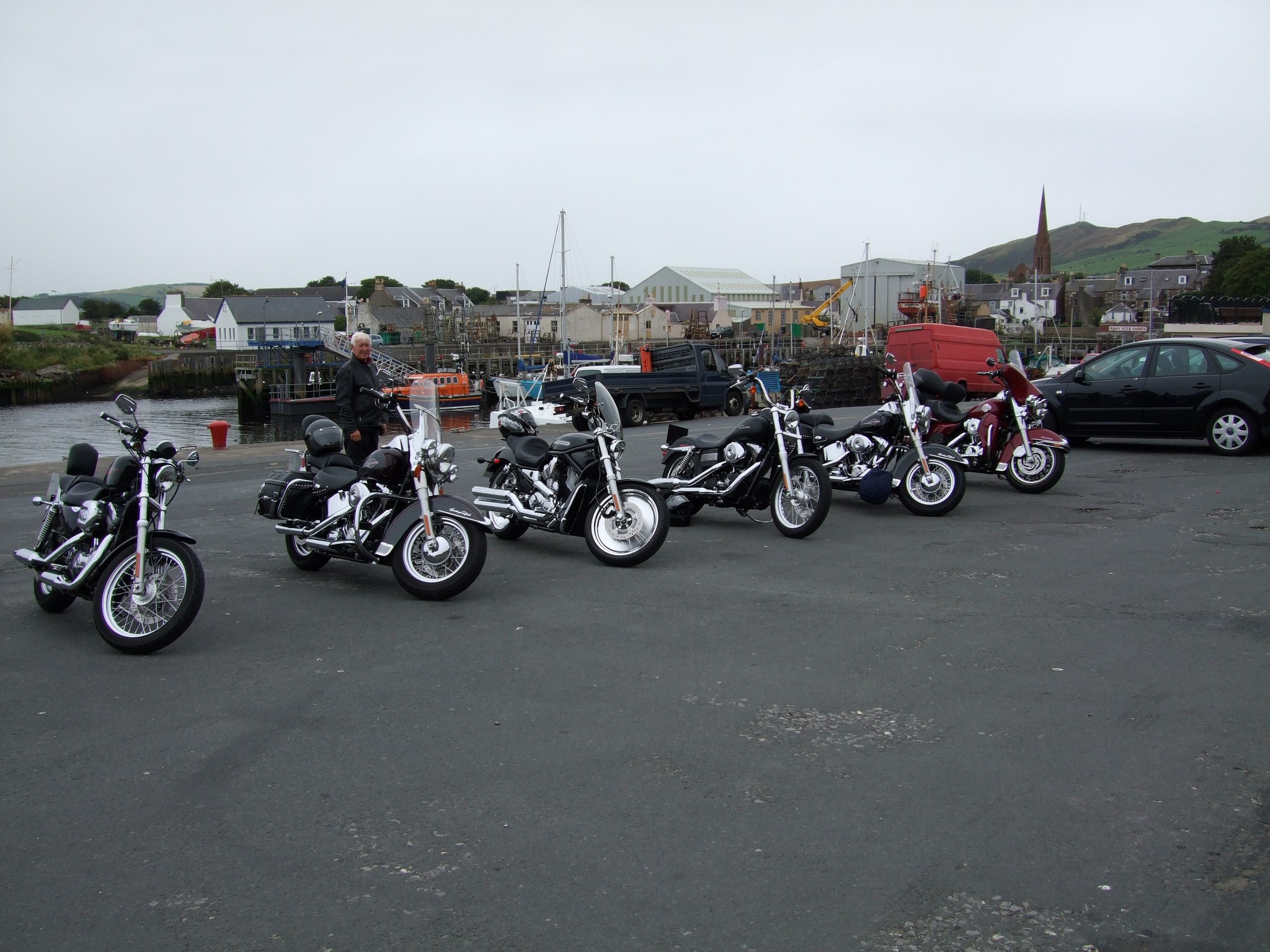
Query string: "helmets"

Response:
xmin=498 ymin=407 xmax=538 ymax=436
xmin=305 ymin=419 xmax=343 ymax=456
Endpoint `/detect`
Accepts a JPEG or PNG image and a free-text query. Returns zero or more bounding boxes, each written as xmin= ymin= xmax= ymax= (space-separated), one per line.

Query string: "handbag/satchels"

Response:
xmin=858 ymin=466 xmax=893 ymax=505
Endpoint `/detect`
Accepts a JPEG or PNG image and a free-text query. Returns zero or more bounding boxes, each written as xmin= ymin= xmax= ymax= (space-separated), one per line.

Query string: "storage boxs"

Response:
xmin=912 ymin=368 xmax=944 ymax=400
xmin=253 ymin=469 xmax=329 ymax=520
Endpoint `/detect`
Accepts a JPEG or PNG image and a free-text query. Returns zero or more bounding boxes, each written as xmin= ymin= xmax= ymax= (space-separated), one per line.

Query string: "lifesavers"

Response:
xmin=304 ymin=355 xmax=312 ymax=364
xmin=480 ymin=379 xmax=484 ymax=389
xmin=1138 ymin=336 xmax=1144 ymax=341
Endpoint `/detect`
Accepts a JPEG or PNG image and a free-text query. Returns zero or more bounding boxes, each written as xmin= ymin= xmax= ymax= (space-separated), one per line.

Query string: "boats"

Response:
xmin=382 ymin=343 xmax=484 ymax=411
xmin=107 ymin=318 xmax=138 ymax=332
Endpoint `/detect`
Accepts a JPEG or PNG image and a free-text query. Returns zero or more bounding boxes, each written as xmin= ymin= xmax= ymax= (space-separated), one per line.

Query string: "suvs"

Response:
xmin=710 ymin=325 xmax=734 ymax=340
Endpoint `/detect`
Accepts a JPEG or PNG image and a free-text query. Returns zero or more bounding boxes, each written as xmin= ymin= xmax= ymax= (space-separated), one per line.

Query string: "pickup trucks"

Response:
xmin=541 ymin=340 xmax=744 ymax=433
xmin=1024 ymin=353 xmax=1108 ymax=381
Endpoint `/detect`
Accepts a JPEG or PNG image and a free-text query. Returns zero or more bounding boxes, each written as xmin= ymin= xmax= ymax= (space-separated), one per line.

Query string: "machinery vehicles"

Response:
xmin=797 ymin=277 xmax=856 ymax=337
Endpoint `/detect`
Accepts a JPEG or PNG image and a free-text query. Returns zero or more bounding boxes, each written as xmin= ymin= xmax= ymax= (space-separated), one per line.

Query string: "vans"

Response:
xmin=574 ymin=365 xmax=641 ymax=378
xmin=881 ymin=322 xmax=1009 ymax=405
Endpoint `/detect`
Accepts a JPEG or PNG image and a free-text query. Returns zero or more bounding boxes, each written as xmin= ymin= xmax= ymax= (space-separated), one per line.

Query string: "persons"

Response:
xmin=336 ymin=332 xmax=388 ymax=465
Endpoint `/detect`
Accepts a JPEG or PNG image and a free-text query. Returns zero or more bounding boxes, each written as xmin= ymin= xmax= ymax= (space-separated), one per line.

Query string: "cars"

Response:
xmin=1130 ymin=337 xmax=1270 ymax=375
xmin=990 ymin=337 xmax=1270 ymax=457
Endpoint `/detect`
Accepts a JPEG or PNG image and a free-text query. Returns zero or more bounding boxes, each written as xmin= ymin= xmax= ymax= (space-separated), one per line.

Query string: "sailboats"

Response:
xmin=487 ymin=208 xmax=649 ymax=402
xmin=896 ymin=241 xmax=965 ymax=318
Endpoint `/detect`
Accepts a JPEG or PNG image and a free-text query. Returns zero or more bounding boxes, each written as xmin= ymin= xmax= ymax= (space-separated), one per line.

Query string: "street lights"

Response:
xmin=317 ymin=311 xmax=323 ymax=344
xmin=355 ymin=299 xmax=362 ymax=331
xmin=52 ymin=290 xmax=63 ymax=331
xmin=263 ymin=299 xmax=269 ymax=346
xmin=741 ymin=309 xmax=745 ymax=338
xmin=436 ymin=298 xmax=442 ymax=358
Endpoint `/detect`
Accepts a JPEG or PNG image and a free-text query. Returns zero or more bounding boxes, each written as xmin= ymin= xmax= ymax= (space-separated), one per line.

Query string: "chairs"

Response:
xmin=1156 ymin=354 xmax=1173 ymax=376
xmin=1172 ymin=353 xmax=1188 ymax=375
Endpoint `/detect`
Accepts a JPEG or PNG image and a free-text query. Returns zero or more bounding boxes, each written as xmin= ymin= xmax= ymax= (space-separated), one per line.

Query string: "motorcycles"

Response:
xmin=254 ymin=378 xmax=491 ymax=601
xmin=472 ymin=377 xmax=670 ymax=567
xmin=13 ymin=393 xmax=206 ymax=654
xmin=658 ymin=364 xmax=833 ymax=539
xmin=780 ymin=352 xmax=970 ymax=517
xmin=883 ymin=350 xmax=1070 ymax=494
